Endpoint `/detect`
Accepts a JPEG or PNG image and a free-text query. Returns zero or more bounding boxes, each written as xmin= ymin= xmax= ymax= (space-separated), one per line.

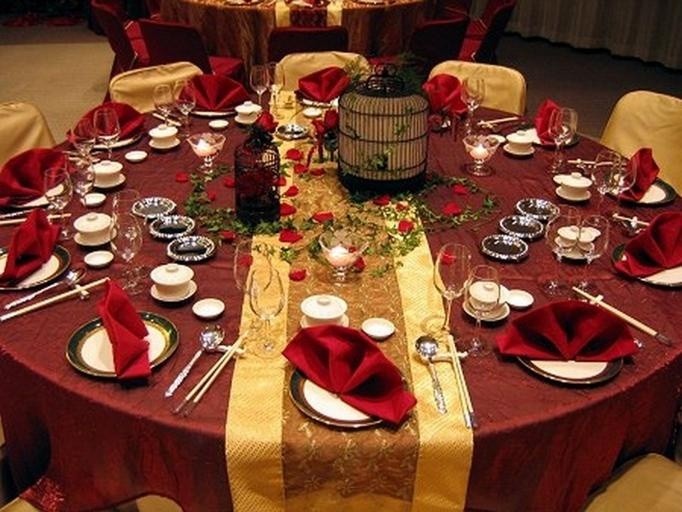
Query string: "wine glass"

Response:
xmin=186 ymin=132 xmax=226 ymax=176
xmin=463 ymin=79 xmax=636 ymax=299
xmin=46 ymin=106 xmax=152 ymax=296
xmin=424 ymin=241 xmax=472 ymax=348
xmin=460 ymin=263 xmax=500 ymax=362
xmin=318 ymin=230 xmax=374 ymax=289
xmin=152 ymin=57 xmax=290 ymax=135
xmin=230 ymin=238 xmax=292 ymax=357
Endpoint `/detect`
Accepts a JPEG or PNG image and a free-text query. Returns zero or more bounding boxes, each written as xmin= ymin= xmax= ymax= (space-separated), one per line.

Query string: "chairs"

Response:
xmin=447 ymin=1 xmax=518 ymax=63
xmin=140 ymin=18 xmax=245 ymax=84
xmin=91 ymin=1 xmax=166 ymax=85
xmin=270 ymin=26 xmax=348 ymax=53
xmin=0 ymin=101 xmax=60 ymax=170
xmin=596 ymin=89 xmax=681 ymax=200
xmin=428 ymin=60 xmax=530 ymax=118
xmin=371 ymin=17 xmax=469 ymax=66
xmin=107 ymin=61 xmax=202 ymax=112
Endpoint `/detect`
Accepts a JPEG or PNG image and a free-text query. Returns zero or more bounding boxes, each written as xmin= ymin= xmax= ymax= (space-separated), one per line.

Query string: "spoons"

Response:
xmin=4 ymin=267 xmax=94 ymax=309
xmin=163 ymin=324 xmax=225 ymax=399
xmin=418 ymin=335 xmax=451 ymax=414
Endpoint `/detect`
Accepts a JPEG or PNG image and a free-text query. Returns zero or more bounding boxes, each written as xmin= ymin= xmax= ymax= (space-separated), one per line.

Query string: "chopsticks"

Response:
xmin=172 ymin=326 xmax=255 ymax=415
xmin=1 ymin=218 xmax=26 ymax=225
xmin=566 ymin=284 xmax=672 ymax=347
xmin=445 ymin=337 xmax=483 ymax=432
xmin=1 ymin=276 xmax=115 ymax=323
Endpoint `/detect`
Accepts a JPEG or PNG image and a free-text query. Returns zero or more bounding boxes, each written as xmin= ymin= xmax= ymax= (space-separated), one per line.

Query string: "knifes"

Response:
xmin=1 ymin=208 xmax=35 ymax=221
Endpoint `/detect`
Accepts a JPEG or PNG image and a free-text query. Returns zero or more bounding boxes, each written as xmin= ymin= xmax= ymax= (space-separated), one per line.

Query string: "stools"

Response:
xmin=576 ymin=451 xmax=680 ymax=512
xmin=1 ymin=476 xmax=184 ymax=510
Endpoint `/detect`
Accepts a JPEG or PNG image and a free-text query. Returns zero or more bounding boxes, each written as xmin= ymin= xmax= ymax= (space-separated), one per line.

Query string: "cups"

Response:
xmin=151 ymin=262 xmax=195 ymax=298
xmin=475 ymin=285 xmax=510 ymax=309
xmin=298 ymin=293 xmax=349 ymax=325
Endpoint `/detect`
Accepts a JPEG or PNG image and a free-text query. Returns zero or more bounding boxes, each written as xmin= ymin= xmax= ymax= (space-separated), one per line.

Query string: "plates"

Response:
xmin=274 ymin=123 xmax=310 ymax=140
xmin=302 ymin=99 xmax=331 ymax=108
xmin=1 ymin=240 xmax=72 ymax=293
xmin=129 ymin=197 xmax=217 ymax=261
xmin=63 ymin=312 xmax=180 ymax=384
xmin=227 ymin=1 xmax=262 ymax=6
xmin=300 ymin=314 xmax=349 ymax=329
xmin=150 ymin=280 xmax=200 ymax=304
xmin=505 ymin=289 xmax=534 ymax=309
xmin=601 ymin=168 xmax=679 ymax=206
xmin=362 ymin=318 xmax=396 ymax=339
xmin=190 ymin=297 xmax=228 ymax=322
xmin=302 ymin=108 xmax=322 ymax=118
xmin=607 ymin=241 xmax=681 ymax=290
xmin=287 ymin=364 xmax=382 ymax=428
xmin=8 ymin=185 xmax=64 ymax=209
xmin=460 ymin=301 xmax=511 ymax=324
xmin=514 ymin=355 xmax=626 ymax=388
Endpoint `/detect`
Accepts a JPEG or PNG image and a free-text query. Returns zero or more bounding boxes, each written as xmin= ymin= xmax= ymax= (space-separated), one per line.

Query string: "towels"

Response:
xmin=616 ymin=148 xmax=661 ymax=203
xmin=616 ymin=208 xmax=680 ymax=280
xmin=491 ymin=300 xmax=641 ymax=363
xmin=293 ymin=66 xmax=352 ymax=103
xmin=281 ymin=323 xmax=417 ymax=427
xmin=95 ymin=276 xmax=152 ymax=379
xmin=177 ymin=73 xmax=253 ymax=112
xmin=1 ymin=146 xmax=80 ymax=204
xmin=532 ymin=98 xmax=563 ymax=148
xmin=1 ymin=209 xmax=62 ymax=284
xmin=66 ymin=101 xmax=149 ymax=142
xmin=424 ymin=74 xmax=469 ymax=116
